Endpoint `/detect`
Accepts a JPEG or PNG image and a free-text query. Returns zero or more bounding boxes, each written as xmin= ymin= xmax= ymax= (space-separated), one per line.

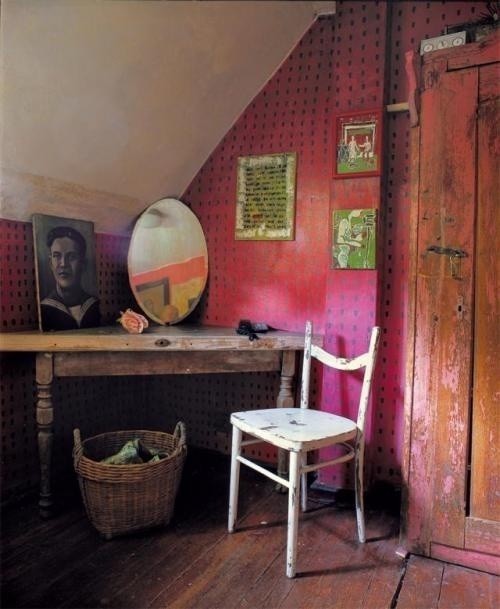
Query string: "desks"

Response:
xmin=0 ymin=322 xmax=325 ymax=518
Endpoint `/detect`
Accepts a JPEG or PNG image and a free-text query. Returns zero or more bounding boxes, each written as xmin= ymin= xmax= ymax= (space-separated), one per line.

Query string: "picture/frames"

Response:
xmin=135 ymin=275 xmax=170 ymax=319
xmin=332 ymin=107 xmax=383 ymax=180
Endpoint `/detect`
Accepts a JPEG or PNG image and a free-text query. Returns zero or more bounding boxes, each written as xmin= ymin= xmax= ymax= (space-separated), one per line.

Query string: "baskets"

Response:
xmin=73 ymin=421 xmax=186 ymax=540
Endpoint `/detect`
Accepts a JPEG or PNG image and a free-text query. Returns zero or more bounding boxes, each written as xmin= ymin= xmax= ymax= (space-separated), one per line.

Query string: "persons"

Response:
xmin=41 ymin=226 xmax=98 ymax=332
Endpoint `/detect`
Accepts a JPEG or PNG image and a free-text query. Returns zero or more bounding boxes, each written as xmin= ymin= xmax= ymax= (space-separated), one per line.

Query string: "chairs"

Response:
xmin=228 ymin=318 xmax=382 ymax=578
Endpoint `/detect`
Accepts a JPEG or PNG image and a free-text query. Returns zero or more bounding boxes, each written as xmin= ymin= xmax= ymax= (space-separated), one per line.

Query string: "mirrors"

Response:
xmin=127 ymin=196 xmax=209 ymax=327
